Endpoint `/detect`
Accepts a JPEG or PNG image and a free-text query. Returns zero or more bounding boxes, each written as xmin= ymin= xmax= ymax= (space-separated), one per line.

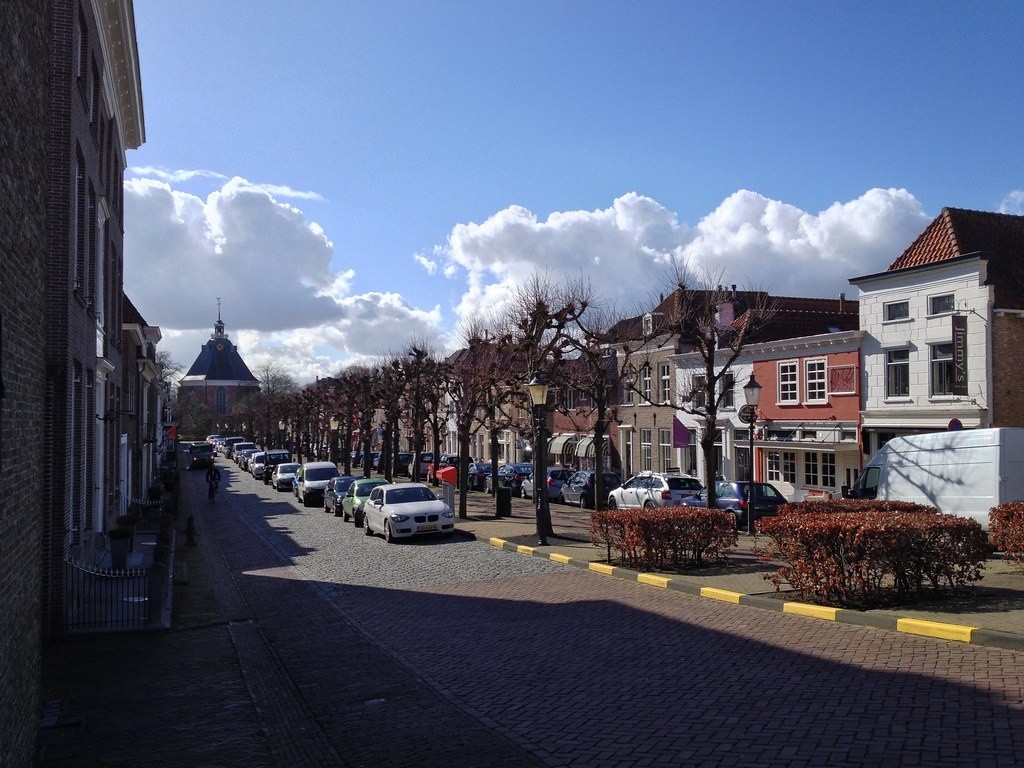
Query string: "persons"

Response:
xmin=206 ymin=462 xmax=221 ymax=500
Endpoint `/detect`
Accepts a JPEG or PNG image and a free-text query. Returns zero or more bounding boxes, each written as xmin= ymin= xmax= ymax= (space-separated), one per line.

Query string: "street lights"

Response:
xmin=528 ymin=370 xmax=554 ymax=545
xmin=743 ymin=372 xmax=762 ymax=535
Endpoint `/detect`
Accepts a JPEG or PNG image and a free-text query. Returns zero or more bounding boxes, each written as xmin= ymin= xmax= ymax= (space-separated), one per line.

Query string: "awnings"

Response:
xmin=544 ymin=434 xmax=608 ymax=459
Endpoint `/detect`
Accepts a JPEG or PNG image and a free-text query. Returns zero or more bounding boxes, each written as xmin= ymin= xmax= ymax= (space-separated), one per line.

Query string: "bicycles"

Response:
xmin=207 ymin=480 xmax=220 ymax=505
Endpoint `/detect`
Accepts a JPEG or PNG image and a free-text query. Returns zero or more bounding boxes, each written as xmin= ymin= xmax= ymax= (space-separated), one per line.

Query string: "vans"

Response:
xmin=297 ymin=461 xmax=342 ymax=507
xmin=839 ymin=427 xmax=1024 ymax=555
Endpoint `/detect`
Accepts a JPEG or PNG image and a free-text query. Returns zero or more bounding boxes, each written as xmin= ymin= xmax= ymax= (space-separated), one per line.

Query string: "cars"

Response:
xmin=342 ymin=478 xmax=399 ymax=528
xmin=559 ymin=470 xmax=623 ymax=509
xmin=190 ymin=435 xmax=300 ymax=497
xmin=679 ymin=481 xmax=788 ymax=532
xmin=363 ymin=482 xmax=454 ymax=543
xmin=352 ymin=448 xmax=576 ymax=504
xmin=608 ymin=470 xmax=704 ymax=512
xmin=324 ymin=476 xmax=366 ymax=517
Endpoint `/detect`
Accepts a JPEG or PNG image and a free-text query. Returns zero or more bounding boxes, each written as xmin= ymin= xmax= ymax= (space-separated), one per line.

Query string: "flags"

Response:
xmin=672 ymin=413 xmax=692 ymax=449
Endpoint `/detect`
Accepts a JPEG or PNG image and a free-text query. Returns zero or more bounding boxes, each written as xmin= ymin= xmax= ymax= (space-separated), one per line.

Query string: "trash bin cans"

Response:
xmin=494 ymin=486 xmax=512 ymax=517
